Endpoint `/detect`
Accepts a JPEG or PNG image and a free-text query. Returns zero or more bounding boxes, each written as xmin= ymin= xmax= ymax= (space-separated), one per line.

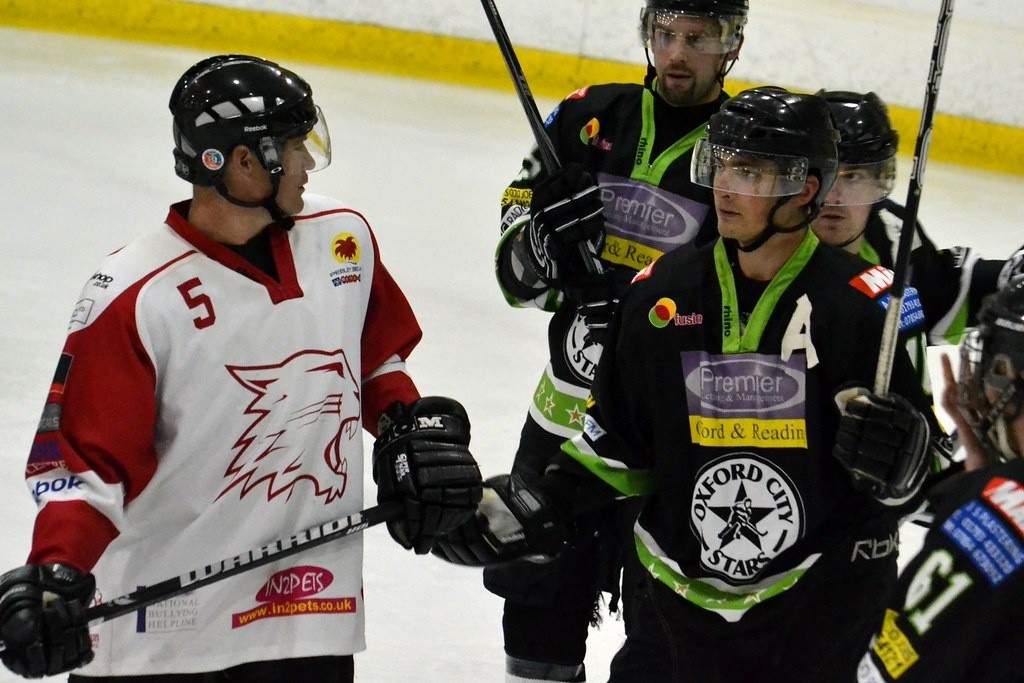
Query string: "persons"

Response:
xmin=435 ymin=1 xmax=1024 ymax=683
xmin=2 ymin=55 xmax=483 ymax=683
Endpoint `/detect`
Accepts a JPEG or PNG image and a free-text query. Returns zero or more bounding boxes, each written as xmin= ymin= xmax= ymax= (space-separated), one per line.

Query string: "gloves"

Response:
xmin=831 ymin=386 xmax=932 ymax=499
xmin=371 ymin=395 xmax=483 ymax=555
xmin=1 ymin=563 xmax=95 ymax=677
xmin=429 ymin=470 xmax=571 ymax=566
xmin=529 ymin=164 xmax=607 ymax=277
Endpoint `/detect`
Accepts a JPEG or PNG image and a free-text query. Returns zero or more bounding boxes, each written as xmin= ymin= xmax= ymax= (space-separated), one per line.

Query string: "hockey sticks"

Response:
xmin=85 ymin=502 xmax=386 ymax=627
xmin=481 ymin=2 xmax=612 ymax=308
xmin=837 ymin=0 xmax=959 ymax=509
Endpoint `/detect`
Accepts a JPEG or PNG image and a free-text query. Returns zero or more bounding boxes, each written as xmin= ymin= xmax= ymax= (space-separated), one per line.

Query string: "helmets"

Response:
xmin=709 ymin=87 xmax=840 ymax=169
xmin=642 ymin=1 xmax=749 ymax=56
xmin=976 ymin=280 xmax=1024 ymax=416
xmin=168 ymin=56 xmax=315 ymax=188
xmin=811 ymin=87 xmax=896 ymax=163
